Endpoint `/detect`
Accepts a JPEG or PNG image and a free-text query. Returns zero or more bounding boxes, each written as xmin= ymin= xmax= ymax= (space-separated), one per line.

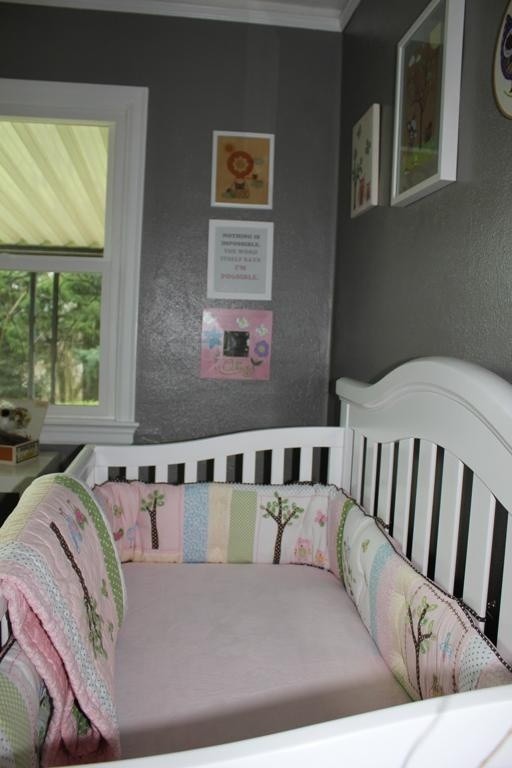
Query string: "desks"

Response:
xmin=0 ymin=451 xmax=58 ymax=500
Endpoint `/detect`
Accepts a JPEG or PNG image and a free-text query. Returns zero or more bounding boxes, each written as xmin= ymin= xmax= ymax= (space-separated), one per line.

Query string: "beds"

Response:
xmin=0 ymin=355 xmax=510 ymax=768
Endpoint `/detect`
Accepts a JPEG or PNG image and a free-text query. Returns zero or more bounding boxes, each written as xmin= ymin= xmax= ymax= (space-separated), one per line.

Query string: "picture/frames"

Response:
xmin=390 ymin=0 xmax=465 ymax=209
